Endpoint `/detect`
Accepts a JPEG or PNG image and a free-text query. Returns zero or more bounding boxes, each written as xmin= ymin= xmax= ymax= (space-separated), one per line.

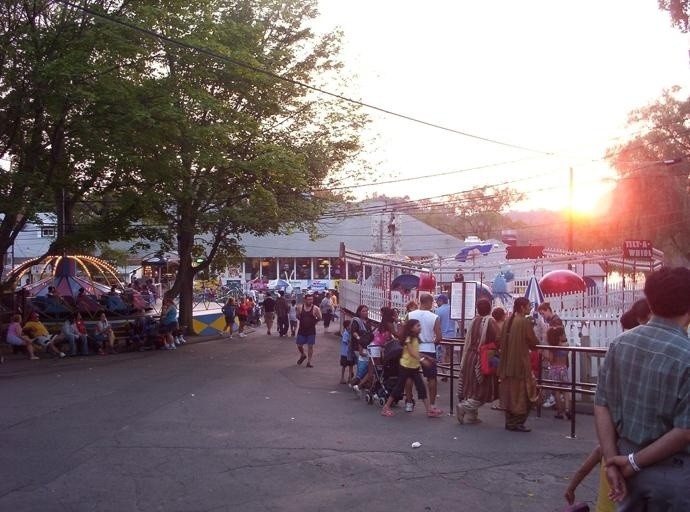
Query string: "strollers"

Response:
xmin=365 ymin=344 xmax=403 ymax=409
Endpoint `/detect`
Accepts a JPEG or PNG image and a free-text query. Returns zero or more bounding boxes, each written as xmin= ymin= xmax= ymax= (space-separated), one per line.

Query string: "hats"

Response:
xmin=31 ymin=312 xmax=39 ymax=320
xmin=435 ymin=294 xmax=448 ymax=302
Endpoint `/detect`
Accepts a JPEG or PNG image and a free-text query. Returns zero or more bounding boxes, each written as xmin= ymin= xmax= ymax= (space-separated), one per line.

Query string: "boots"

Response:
xmin=456 ymin=404 xmax=482 ymax=424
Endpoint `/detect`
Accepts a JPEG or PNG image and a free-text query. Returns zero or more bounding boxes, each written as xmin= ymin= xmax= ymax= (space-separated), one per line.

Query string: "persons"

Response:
xmin=7 ymin=263 xmax=186 ymax=360
xmin=619 ymin=310 xmax=639 ymax=333
xmin=338 ymin=293 xmax=571 ymax=434
xmin=195 ymin=261 xmax=341 ymax=368
xmin=629 ymin=297 xmax=652 ymax=325
xmin=592 ymin=263 xmax=689 ymax=511
xmin=562 ymin=439 xmax=618 ymax=512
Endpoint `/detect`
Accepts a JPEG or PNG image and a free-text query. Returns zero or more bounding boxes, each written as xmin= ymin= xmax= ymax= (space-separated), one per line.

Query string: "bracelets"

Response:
xmin=627 ymin=451 xmax=642 ymax=473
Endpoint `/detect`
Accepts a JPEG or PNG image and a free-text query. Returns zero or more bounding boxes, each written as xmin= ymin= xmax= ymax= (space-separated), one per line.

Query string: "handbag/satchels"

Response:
xmin=384 ymin=340 xmax=404 ymax=363
xmin=479 ymin=342 xmax=499 ymax=377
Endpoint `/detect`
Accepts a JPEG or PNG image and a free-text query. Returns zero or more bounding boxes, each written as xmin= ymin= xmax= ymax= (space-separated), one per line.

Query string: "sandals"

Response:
xmin=565 ymin=408 xmax=569 ymax=419
xmin=555 ymin=411 xmax=564 ymax=419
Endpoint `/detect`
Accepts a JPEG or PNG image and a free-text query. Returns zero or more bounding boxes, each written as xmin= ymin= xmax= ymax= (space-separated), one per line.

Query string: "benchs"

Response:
xmin=0 ymin=316 xmax=188 ymax=364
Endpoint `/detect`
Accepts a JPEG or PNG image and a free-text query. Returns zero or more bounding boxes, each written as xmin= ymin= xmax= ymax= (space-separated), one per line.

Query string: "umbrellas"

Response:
xmin=454 ymin=244 xmax=494 ymax=265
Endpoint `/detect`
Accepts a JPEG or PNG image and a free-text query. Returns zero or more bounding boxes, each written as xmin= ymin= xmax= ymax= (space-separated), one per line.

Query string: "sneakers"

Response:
xmin=164 ymin=335 xmax=186 ymax=350
xmin=30 ymin=357 xmax=39 ymax=360
xmin=59 ymin=352 xmax=66 ymax=358
xmin=353 ymin=385 xmax=360 ymax=398
xmin=240 ymin=333 xmax=245 ymax=337
xmin=505 ymin=422 xmax=532 ymax=432
xmin=382 ymin=399 xmax=443 ymax=418
